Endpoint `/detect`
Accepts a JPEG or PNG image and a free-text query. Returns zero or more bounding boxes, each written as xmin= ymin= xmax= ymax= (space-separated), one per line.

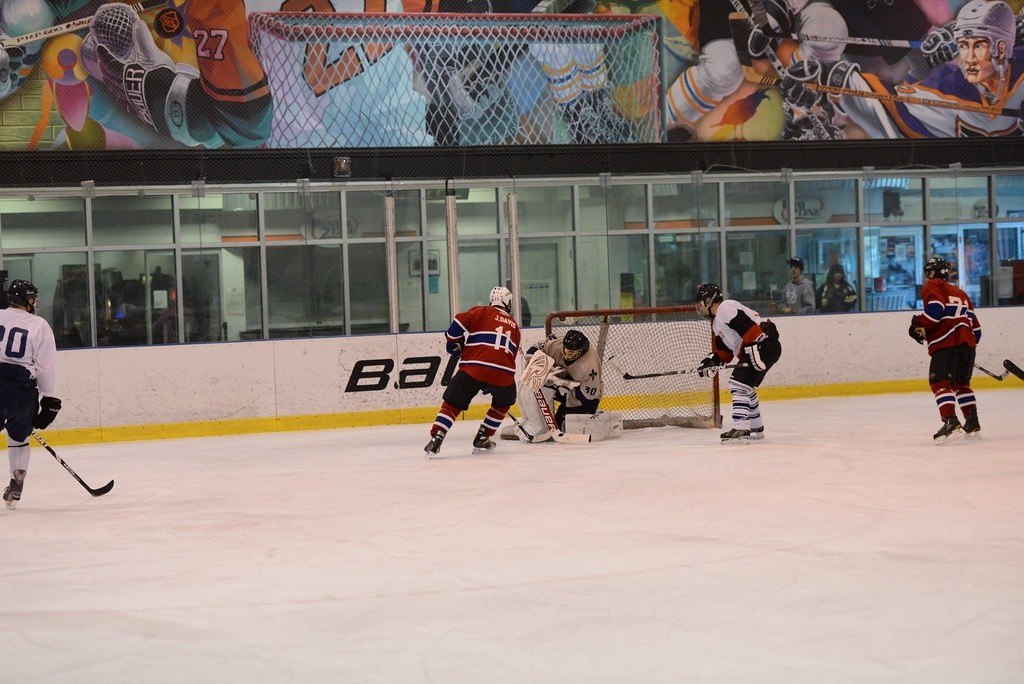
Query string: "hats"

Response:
xmin=787 ymin=256 xmax=804 ymax=267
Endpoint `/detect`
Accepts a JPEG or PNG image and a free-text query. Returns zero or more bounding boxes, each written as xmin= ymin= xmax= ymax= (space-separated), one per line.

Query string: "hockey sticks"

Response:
xmin=519 ymin=345 xmax=591 ymax=443
xmin=608 ymin=356 xmax=749 ymax=380
xmin=1003 ymin=359 xmax=1024 ymax=381
xmin=30 ymin=430 xmax=114 ymax=497
xmin=507 ymin=409 xmax=554 ymax=442
xmin=973 ymin=363 xmax=1010 ymax=381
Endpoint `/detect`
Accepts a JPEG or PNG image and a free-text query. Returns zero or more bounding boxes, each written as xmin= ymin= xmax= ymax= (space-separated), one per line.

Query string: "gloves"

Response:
xmin=446 ymin=340 xmax=464 ymax=358
xmin=760 ymin=319 xmax=779 ymax=339
xmin=697 ymin=352 xmax=723 ymax=378
xmin=34 ymin=396 xmax=61 ymax=430
xmin=909 ymin=315 xmax=925 ymax=344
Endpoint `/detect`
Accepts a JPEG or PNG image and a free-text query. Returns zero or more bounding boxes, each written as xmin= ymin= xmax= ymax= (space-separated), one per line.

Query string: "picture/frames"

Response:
xmin=408 ymin=250 xmax=440 ymax=277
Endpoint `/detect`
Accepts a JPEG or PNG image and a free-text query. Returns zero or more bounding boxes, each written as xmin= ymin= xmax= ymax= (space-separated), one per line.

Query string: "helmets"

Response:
xmin=924 ymin=257 xmax=950 ymax=281
xmin=563 ymin=330 xmax=590 ymax=366
xmin=8 ymin=279 xmax=39 ymax=315
xmin=693 ymin=283 xmax=722 ymax=318
xmin=490 ymin=287 xmax=513 ymax=314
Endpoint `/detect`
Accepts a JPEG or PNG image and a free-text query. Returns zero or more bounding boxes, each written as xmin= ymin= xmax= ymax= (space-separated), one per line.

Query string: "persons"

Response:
xmin=776 ymin=256 xmax=815 ymax=315
xmin=424 ymin=286 xmax=521 ymax=460
xmin=0 ymin=279 xmax=62 ymax=510
xmin=815 ymin=264 xmax=855 ymax=314
xmin=908 ymin=258 xmax=982 ymax=445
xmin=696 ymin=284 xmax=781 ymax=445
xmin=489 ymin=280 xmax=531 ymax=327
xmin=500 ymin=329 xmax=604 ymax=440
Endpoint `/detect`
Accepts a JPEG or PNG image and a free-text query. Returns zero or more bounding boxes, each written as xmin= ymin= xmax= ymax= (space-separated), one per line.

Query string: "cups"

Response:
xmin=770 ymin=302 xmax=776 ymax=311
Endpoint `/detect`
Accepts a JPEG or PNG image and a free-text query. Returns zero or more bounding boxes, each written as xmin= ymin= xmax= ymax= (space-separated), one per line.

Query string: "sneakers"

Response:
xmin=962 ymin=407 xmax=981 ymax=439
xmin=472 ymin=424 xmax=497 ymax=453
xmin=424 ymin=430 xmax=443 ymax=459
xmin=934 ymin=413 xmax=966 ymax=444
xmin=4 ymin=469 xmax=26 ymax=507
xmin=720 ymin=428 xmax=750 ymax=445
xmin=749 ymin=426 xmax=764 ymax=439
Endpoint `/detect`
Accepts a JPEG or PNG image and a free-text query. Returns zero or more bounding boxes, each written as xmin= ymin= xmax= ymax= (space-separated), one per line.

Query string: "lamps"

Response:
xmin=334 ymin=156 xmax=351 ymax=177
xmin=192 ymin=181 xmax=204 ymax=196
xmin=691 ymin=170 xmax=703 ymax=185
xmin=81 ymin=179 xmax=95 ymax=199
xmin=599 ymin=172 xmax=611 ymax=189
xmin=863 ymin=166 xmax=873 ymax=181
xmin=782 ymin=167 xmax=792 ymax=184
xmin=950 ymin=162 xmax=962 ymax=178
xmin=296 ymin=179 xmax=311 ymax=196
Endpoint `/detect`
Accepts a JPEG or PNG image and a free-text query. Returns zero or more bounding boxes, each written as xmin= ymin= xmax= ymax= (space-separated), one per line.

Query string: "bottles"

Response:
xmin=874 ymin=277 xmax=886 ymax=291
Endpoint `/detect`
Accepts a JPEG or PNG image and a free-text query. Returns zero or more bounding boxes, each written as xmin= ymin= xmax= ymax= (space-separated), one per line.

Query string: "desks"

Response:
xmin=120 ymin=309 xmax=194 ymax=344
xmin=866 ymin=289 xmax=904 ymax=311
